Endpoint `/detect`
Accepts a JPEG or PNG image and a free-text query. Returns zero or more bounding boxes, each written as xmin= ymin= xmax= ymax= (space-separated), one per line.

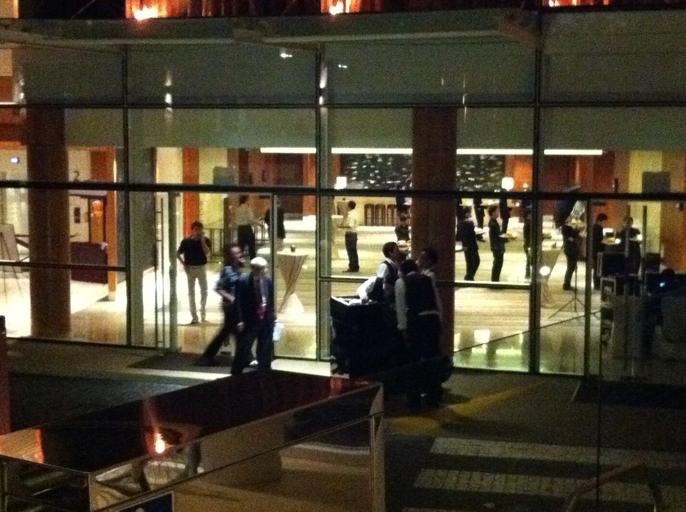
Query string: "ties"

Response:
xmin=255 ymin=276 xmax=266 ymax=319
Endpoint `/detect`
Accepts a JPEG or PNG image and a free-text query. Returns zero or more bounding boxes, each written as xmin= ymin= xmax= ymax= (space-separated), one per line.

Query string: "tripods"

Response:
xmin=544 ymin=221 xmax=600 ymax=322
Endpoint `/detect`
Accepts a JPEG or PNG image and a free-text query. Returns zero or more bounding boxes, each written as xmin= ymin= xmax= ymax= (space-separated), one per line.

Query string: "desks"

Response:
xmin=337 ymin=200 xmax=348 ymax=228
xmin=204 ymin=216 xmax=265 ymax=263
xmin=331 ymin=214 xmax=344 ymax=259
xmin=528 ymin=228 xmax=561 ymax=308
xmin=276 ymin=250 xmax=309 ymax=315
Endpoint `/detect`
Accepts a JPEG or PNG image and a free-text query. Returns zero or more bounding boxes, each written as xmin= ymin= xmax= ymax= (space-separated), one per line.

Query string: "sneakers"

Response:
xmin=190 ymin=317 xmax=198 ymax=325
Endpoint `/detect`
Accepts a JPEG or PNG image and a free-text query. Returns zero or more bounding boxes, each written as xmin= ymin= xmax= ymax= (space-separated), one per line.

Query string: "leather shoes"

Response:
xmin=342 ymin=268 xmax=359 ymax=273
xmin=564 ymin=285 xmax=577 ymax=291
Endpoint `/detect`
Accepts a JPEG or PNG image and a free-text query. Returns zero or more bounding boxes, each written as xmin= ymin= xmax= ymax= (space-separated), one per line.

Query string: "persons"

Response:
xmin=198 ymin=244 xmax=247 ymax=366
xmin=619 ymin=216 xmax=641 ymax=273
xmin=456 ymin=198 xmax=532 ymax=282
xmin=336 ymin=201 xmax=359 ymax=272
xmin=653 ymin=269 xmax=680 ymax=293
xmin=234 ymin=196 xmax=256 ymax=260
xmin=265 ymin=198 xmax=286 ymax=251
xmin=176 ymin=221 xmax=213 ymax=325
xmin=394 ymin=179 xmax=412 ymax=241
xmin=593 ymin=213 xmax=608 ymax=289
xmin=355 ymin=242 xmax=444 ymax=407
xmin=559 ymin=211 xmax=585 ymax=290
xmin=231 ymin=258 xmax=275 ymax=375
